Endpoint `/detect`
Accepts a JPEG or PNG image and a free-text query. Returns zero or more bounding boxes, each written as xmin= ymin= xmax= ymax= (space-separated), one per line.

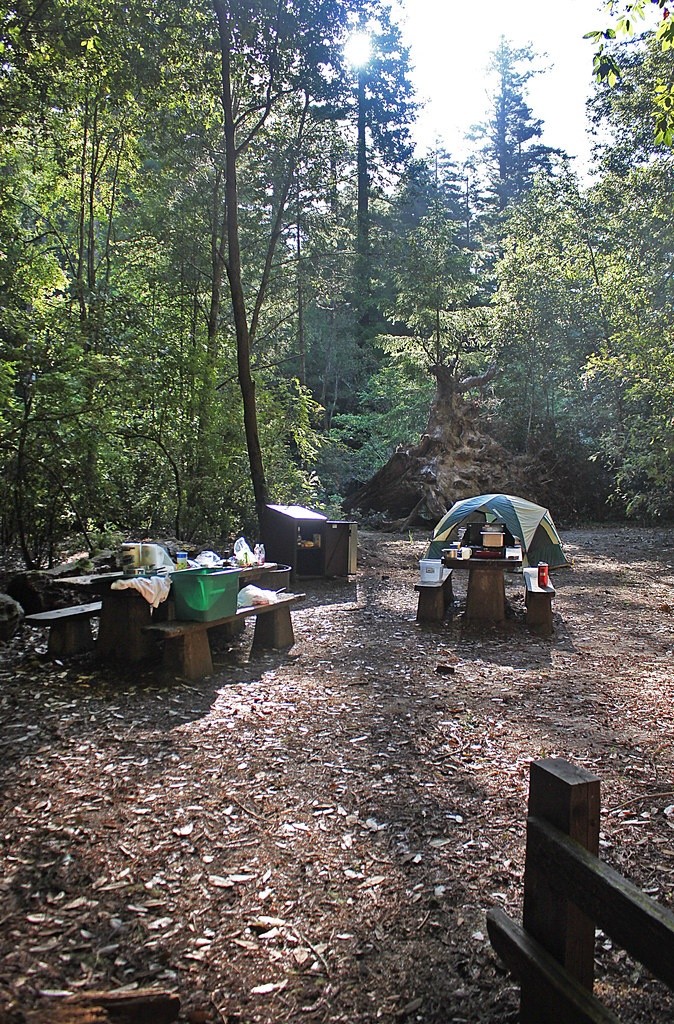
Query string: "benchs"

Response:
xmin=414 ymin=566 xmax=456 ymax=627
xmin=25 ymin=599 xmax=103 ymax=657
xmin=522 ymin=565 xmax=555 ymax=629
xmin=144 ymin=588 xmax=307 ymax=681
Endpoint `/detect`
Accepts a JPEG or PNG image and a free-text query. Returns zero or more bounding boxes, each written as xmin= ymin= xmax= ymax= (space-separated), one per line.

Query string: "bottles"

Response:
xmin=254 ymin=543 xmax=261 ymax=566
xmin=260 ymin=543 xmax=266 ymax=566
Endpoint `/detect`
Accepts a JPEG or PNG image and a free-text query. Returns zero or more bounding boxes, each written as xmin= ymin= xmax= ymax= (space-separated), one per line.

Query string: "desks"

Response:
xmin=50 ymin=560 xmax=278 ymax=664
xmin=441 ymin=542 xmax=523 ymax=620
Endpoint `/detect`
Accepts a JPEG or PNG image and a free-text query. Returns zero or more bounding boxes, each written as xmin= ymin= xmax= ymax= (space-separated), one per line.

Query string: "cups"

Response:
xmin=176 ymin=552 xmax=188 ymax=571
xmin=461 ymin=547 xmax=472 ymax=560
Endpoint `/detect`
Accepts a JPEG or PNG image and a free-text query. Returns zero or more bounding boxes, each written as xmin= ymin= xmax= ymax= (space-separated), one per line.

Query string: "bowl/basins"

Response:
xmin=442 ymin=549 xmax=457 ymax=560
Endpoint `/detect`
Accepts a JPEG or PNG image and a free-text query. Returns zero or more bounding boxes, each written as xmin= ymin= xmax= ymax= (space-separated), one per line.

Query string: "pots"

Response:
xmin=480 ymin=531 xmax=505 ymax=547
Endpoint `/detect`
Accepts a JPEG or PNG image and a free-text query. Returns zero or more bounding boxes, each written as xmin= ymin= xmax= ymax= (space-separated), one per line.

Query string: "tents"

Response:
xmin=422 ymin=493 xmax=569 ymax=573
